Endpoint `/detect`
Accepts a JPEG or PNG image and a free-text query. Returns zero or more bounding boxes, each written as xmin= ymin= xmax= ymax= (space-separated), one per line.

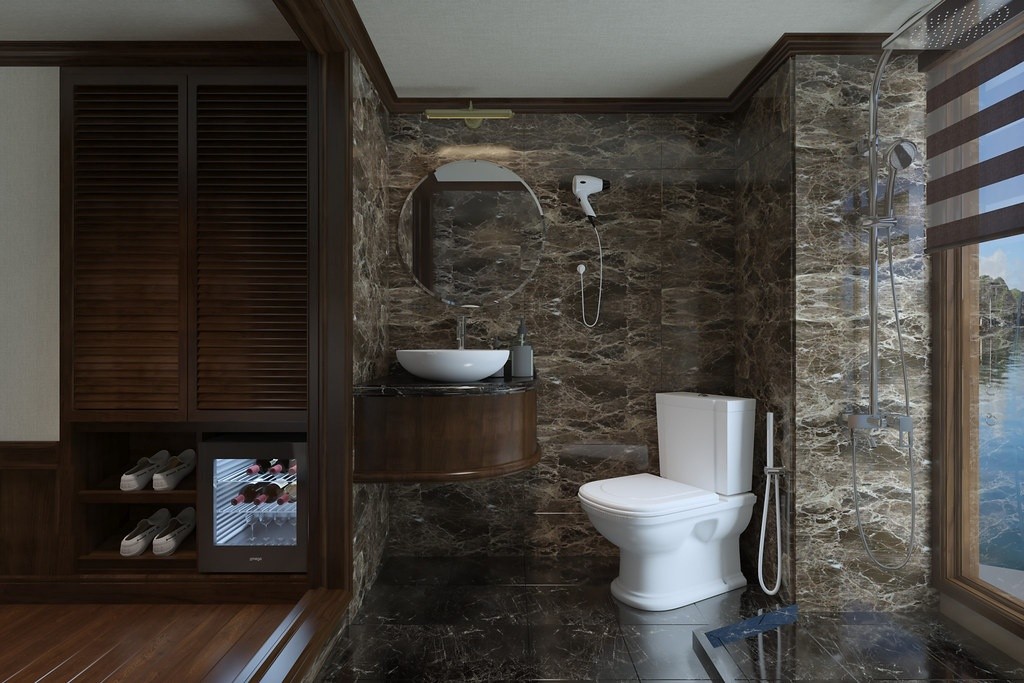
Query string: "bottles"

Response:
xmin=270 ymin=461 xmax=288 ymax=475
xmin=254 ymin=475 xmax=295 ymax=505
xmin=247 ymin=459 xmax=272 ymax=475
xmin=288 ymin=465 xmax=297 ymax=475
xmin=231 ymin=474 xmax=277 ymax=505
xmin=277 ymin=480 xmax=297 ymax=505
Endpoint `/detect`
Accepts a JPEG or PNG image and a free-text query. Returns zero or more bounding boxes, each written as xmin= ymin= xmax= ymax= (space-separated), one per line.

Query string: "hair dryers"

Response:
xmin=573 ymin=173 xmax=614 ymax=229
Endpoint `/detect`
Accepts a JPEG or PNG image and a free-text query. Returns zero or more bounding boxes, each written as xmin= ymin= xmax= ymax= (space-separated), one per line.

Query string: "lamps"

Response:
xmin=425 ymin=101 xmax=515 ymax=119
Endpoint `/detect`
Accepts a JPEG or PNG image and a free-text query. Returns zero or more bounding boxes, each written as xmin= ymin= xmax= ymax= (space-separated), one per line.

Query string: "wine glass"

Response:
xmin=274 ymin=513 xmax=287 ymax=542
xmin=245 ymin=513 xmax=258 ymax=541
xmin=259 ymin=513 xmax=273 ymax=541
xmin=288 ymin=513 xmax=298 ymax=542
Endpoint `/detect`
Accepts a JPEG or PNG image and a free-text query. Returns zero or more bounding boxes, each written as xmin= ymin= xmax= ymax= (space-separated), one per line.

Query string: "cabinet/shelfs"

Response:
xmin=59 ymin=75 xmax=321 ymax=607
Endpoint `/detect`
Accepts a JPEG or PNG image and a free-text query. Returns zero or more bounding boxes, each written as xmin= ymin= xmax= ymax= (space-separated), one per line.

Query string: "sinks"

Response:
xmin=393 ymin=348 xmax=510 ymax=382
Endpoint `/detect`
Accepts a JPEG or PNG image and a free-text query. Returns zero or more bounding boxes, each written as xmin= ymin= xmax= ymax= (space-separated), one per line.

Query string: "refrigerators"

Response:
xmin=196 ymin=440 xmax=310 ymax=571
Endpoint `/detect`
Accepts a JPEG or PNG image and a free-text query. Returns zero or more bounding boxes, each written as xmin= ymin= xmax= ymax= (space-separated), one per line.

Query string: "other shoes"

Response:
xmin=120 ymin=508 xmax=171 ymax=557
xmin=152 ymin=448 xmax=196 ymax=492
xmin=119 ymin=449 xmax=171 ymax=490
xmin=152 ymin=507 xmax=197 ymax=558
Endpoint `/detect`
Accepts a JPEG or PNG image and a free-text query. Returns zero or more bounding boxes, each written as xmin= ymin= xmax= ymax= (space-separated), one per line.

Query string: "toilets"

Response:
xmin=576 ymin=388 xmax=758 ymax=612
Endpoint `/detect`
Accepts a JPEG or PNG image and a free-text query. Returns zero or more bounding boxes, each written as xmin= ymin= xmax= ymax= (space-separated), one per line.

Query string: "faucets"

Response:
xmin=456 ymin=314 xmax=466 ymax=348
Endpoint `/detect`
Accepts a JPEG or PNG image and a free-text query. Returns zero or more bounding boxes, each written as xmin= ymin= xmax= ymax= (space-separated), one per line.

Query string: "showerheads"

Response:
xmin=883 ymin=136 xmax=920 ymax=217
xmin=880 ymin=0 xmax=1024 ymax=53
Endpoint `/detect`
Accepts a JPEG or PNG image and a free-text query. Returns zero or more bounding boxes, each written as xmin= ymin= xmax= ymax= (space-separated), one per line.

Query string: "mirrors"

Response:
xmin=396 ymin=159 xmax=545 ymax=309
